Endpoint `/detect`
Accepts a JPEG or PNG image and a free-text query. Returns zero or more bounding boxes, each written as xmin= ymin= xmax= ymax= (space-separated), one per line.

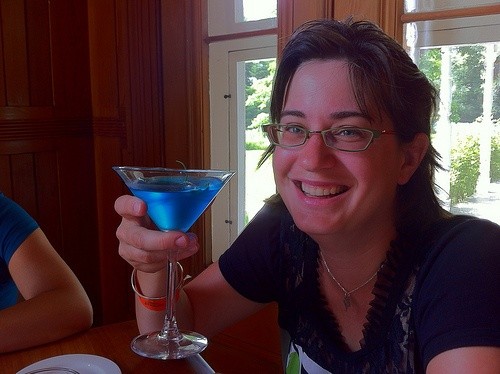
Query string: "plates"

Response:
xmin=15 ymin=354 xmax=122 ymax=374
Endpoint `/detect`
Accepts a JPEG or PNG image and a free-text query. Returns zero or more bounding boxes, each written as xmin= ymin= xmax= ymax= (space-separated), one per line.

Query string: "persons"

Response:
xmin=0 ymin=192 xmax=94 ymax=353
xmin=115 ymin=13 xmax=500 ymax=374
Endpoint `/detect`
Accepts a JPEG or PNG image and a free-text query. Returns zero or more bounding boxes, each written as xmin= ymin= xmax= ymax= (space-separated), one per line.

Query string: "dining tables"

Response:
xmin=0 ymin=320 xmax=214 ymax=374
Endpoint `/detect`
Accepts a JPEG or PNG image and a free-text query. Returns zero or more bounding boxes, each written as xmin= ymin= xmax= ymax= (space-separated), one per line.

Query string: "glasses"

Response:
xmin=261 ymin=123 xmax=398 ymax=151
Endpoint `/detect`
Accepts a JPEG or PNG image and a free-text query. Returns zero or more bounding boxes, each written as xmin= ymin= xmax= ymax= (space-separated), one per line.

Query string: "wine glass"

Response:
xmin=112 ymin=166 xmax=236 ymax=358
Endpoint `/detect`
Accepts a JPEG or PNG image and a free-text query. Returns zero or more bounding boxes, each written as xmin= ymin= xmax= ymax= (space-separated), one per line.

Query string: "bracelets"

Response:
xmin=131 ymin=259 xmax=184 ymax=311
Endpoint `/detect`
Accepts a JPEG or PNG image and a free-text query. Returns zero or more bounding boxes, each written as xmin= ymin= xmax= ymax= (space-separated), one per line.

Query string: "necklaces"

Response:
xmin=319 ymin=242 xmax=386 ymax=312
